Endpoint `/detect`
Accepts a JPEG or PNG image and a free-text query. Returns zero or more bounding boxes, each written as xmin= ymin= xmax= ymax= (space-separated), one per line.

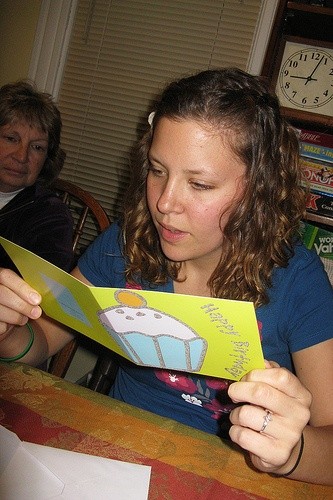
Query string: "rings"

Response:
xmin=260 ymin=412 xmax=272 ymax=433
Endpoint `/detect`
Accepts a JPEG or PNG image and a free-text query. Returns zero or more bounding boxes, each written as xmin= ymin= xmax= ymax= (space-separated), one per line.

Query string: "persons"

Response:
xmin=0 ymin=68 xmax=332 ymax=486
xmin=0 ymin=78 xmax=76 ymax=296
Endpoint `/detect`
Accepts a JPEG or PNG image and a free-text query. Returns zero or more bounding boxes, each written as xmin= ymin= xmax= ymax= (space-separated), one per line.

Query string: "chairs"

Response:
xmin=43 ymin=178 xmax=110 ymax=378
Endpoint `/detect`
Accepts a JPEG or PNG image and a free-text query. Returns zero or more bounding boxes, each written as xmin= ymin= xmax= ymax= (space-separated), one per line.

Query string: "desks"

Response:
xmin=0 ymin=359 xmax=333 ymax=500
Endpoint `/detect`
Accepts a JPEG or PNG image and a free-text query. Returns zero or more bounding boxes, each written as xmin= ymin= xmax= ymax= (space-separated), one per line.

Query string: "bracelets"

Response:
xmin=0 ymin=322 xmax=34 ymax=362
xmin=280 ymin=433 xmax=304 ymax=477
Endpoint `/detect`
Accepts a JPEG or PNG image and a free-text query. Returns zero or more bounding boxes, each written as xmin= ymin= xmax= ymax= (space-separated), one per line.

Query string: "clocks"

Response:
xmin=267 ymin=33 xmax=333 ymax=125
